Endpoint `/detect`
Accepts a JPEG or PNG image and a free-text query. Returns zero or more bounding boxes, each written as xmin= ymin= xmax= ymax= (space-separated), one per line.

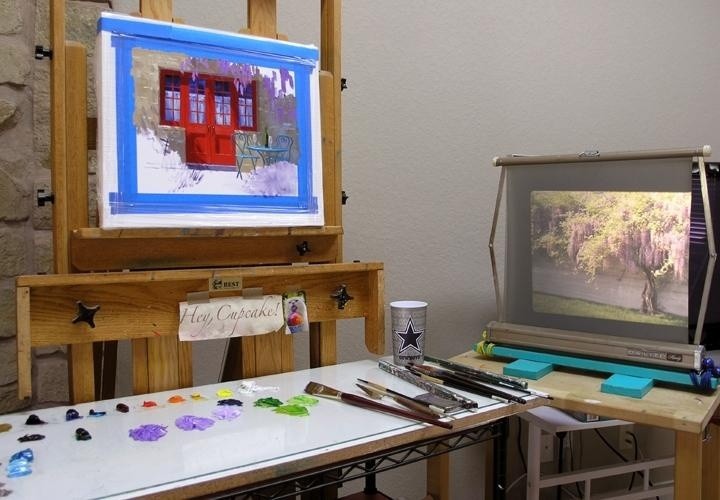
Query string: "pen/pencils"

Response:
xmin=305 ymin=355 xmax=554 ymax=429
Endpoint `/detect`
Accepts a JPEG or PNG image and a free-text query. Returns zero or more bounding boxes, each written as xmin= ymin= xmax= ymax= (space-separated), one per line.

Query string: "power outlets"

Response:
xmin=618 ymin=424 xmax=635 ymax=451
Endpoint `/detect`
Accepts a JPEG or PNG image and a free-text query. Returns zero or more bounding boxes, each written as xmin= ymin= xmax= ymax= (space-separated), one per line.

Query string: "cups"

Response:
xmin=389 ymin=300 xmax=428 ymax=367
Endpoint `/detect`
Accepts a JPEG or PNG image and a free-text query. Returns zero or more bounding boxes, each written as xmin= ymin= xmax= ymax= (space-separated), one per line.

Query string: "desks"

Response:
xmin=2 ymin=355 xmax=552 ymax=500
xmin=427 ymin=345 xmax=719 ymax=500
